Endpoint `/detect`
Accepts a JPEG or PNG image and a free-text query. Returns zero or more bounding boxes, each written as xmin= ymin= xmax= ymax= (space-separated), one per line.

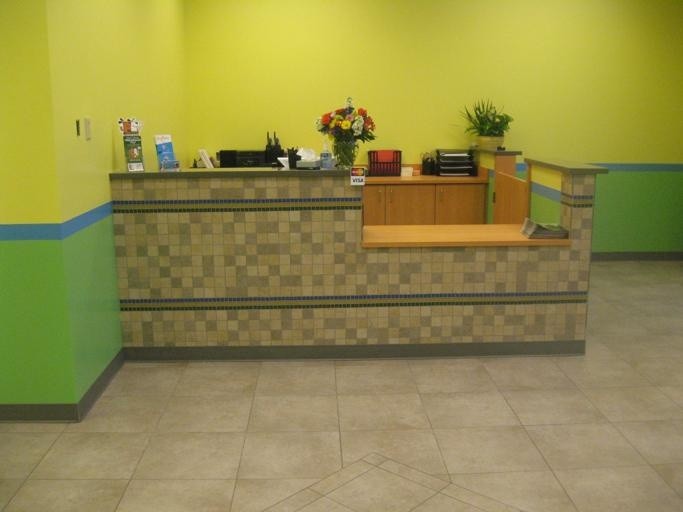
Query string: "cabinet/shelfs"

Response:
xmin=363 ymin=170 xmax=488 ymax=225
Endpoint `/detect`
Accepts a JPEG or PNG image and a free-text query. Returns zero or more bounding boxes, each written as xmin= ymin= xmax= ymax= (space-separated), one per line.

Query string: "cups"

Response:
xmin=420 ymin=157 xmax=436 ymax=175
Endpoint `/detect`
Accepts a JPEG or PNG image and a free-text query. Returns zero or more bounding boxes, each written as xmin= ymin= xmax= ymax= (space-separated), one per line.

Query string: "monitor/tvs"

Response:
xmin=220 ymin=150 xmax=272 ymax=167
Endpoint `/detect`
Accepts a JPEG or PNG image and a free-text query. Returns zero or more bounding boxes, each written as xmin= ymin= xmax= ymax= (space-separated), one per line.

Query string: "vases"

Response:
xmin=332 ymin=136 xmax=360 ymax=168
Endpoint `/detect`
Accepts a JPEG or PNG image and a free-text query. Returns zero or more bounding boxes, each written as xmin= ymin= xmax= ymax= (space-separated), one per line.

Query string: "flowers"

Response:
xmin=315 ymin=97 xmax=377 ymax=168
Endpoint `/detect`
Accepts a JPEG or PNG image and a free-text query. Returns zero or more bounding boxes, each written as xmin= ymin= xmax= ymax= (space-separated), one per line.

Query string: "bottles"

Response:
xmin=320 ymin=143 xmax=333 ymax=170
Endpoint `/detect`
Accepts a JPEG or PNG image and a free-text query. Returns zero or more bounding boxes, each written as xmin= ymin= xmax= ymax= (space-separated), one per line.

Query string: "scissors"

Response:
xmin=420 ymin=152 xmax=434 ymax=163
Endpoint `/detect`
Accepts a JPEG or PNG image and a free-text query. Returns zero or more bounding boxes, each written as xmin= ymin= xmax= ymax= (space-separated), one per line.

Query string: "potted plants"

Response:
xmin=459 ymin=97 xmax=514 ymax=149
